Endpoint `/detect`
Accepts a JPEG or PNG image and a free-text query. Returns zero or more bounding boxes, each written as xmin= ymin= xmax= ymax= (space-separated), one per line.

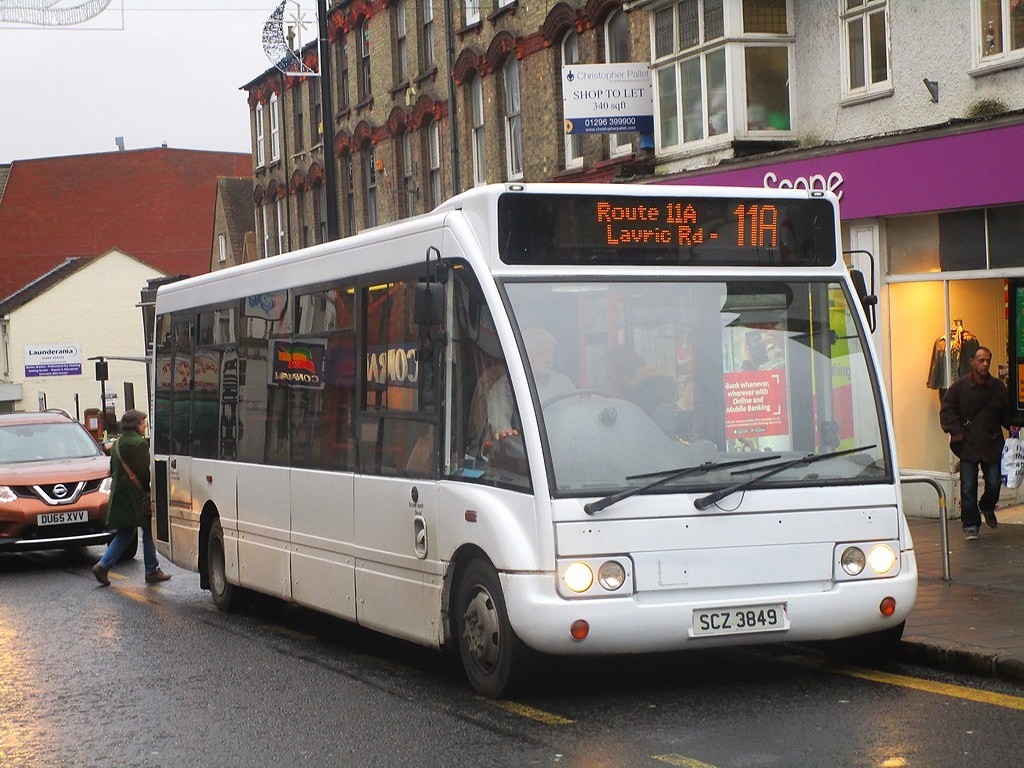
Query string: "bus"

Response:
xmin=144 ymin=183 xmax=918 ymax=692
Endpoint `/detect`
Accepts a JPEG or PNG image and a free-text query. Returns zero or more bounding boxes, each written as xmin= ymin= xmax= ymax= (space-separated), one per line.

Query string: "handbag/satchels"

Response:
xmin=1001 ymin=431 xmax=1024 ymax=489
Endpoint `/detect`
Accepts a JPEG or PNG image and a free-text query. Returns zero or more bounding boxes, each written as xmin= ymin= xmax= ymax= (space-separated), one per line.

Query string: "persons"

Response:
xmin=91 ymin=410 xmax=172 ymax=586
xmin=487 ymin=328 xmax=575 ymax=477
xmin=926 ymin=324 xmax=979 ymax=403
xmin=939 ymin=346 xmax=1010 ymax=539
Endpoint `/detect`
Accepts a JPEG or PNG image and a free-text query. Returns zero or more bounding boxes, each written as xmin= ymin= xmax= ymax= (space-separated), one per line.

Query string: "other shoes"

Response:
xmin=979 ymin=501 xmax=997 ymax=528
xmin=91 ymin=564 xmax=111 ymax=586
xmin=964 ymin=529 xmax=979 ymax=540
xmin=145 ymin=571 xmax=172 ymax=583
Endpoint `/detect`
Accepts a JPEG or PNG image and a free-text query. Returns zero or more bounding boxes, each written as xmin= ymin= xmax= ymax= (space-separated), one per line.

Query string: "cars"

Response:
xmin=0 ymin=408 xmax=138 ymax=558
